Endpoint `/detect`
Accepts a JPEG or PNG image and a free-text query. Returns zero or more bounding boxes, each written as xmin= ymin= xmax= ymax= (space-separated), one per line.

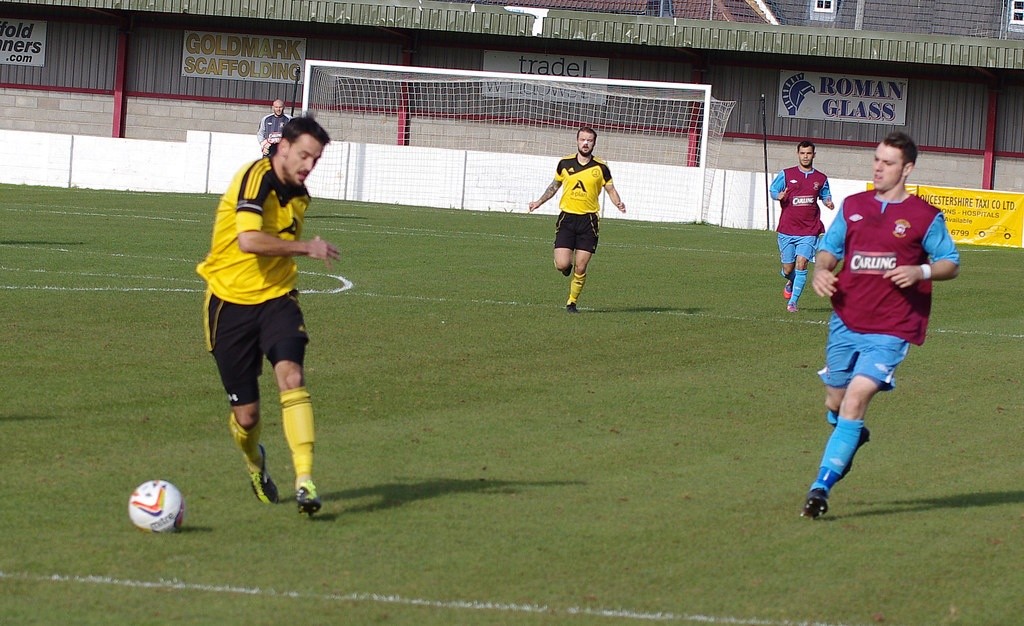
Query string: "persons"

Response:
xmin=255 ymin=99 xmax=294 ymax=157
xmin=194 ymin=117 xmax=323 ymax=514
xmin=528 ymin=127 xmax=626 ymax=311
xmin=769 ymin=139 xmax=834 ymax=314
xmin=801 ymin=132 xmax=961 ymax=520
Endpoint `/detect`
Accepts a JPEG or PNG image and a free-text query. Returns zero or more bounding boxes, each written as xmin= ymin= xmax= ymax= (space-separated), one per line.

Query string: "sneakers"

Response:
xmin=786 ymin=301 xmax=799 ymax=312
xmin=565 ymin=299 xmax=578 ymax=313
xmin=800 ymin=487 xmax=828 ymax=520
xmin=295 ymin=480 xmax=321 ymax=514
xmin=561 ymin=263 xmax=573 ymax=277
xmin=244 ymin=443 xmax=278 ymax=506
xmin=783 ymin=282 xmax=792 ymax=299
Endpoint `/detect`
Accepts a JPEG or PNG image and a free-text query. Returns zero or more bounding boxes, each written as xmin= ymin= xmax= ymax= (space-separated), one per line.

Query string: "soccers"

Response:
xmin=128 ymin=479 xmax=186 ymax=533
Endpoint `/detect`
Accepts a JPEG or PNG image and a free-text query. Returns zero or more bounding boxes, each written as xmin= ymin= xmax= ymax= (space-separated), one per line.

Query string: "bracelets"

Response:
xmin=921 ymin=263 xmax=932 ymax=280
